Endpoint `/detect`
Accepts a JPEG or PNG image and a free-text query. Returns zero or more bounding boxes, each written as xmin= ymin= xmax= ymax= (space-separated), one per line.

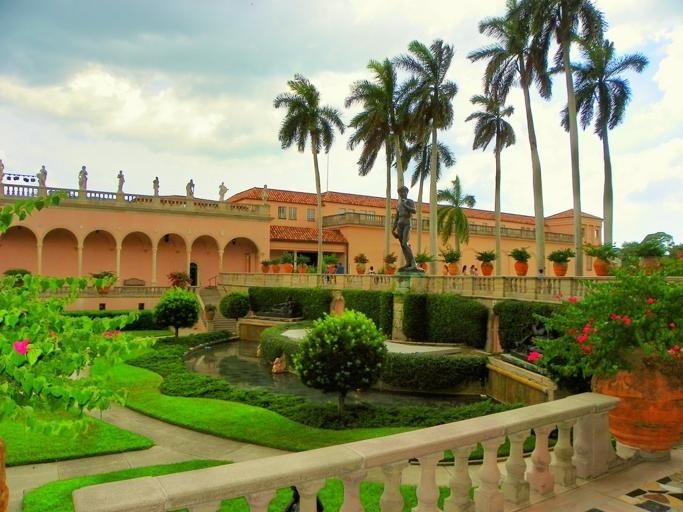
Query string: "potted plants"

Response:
xmin=414 ymin=250 xmax=436 ymax=274
xmin=3 ymin=268 xmax=32 ymax=288
xmin=87 ymin=270 xmax=120 ymax=294
xmin=261 ymin=259 xmax=271 ymax=273
xmin=322 ymin=254 xmax=343 ymax=274
xmin=545 ymin=247 xmax=575 ymax=276
xmin=474 ymin=248 xmax=498 ymax=276
xmin=271 ymin=257 xmax=281 ymax=273
xmin=281 ymin=251 xmax=295 ymax=273
xmin=204 ymin=303 xmax=217 ymax=320
xmin=582 ymin=241 xmax=618 ymax=276
xmin=167 ymin=271 xmax=192 ymax=289
xmin=439 ymin=245 xmax=463 ymax=275
xmin=383 ymin=251 xmax=399 ymax=274
xmin=295 ymin=255 xmax=311 ymax=274
xmin=507 ymin=247 xmax=531 ymax=276
xmin=634 ymin=241 xmax=666 ymax=276
xmin=353 ymin=252 xmax=371 ymax=274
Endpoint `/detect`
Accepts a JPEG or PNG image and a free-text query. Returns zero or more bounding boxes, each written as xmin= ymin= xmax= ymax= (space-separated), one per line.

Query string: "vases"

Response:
xmin=0 ymin=440 xmax=13 ymax=512
xmin=591 ymin=347 xmax=683 ymax=451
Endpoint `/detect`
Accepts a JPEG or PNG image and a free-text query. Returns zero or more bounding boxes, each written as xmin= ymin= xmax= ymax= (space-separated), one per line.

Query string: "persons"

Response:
xmin=152 ymin=177 xmax=159 ymax=196
xmin=186 ymin=179 xmax=194 ymax=196
xmin=38 ymin=165 xmax=47 ymax=188
xmin=470 ymin=265 xmax=475 ymax=271
xmin=442 ymin=264 xmax=448 ymax=274
xmin=335 ymin=262 xmax=344 ymax=274
xmin=332 ymin=265 xmax=337 ymax=274
xmin=536 ymin=269 xmax=544 ymax=276
xmin=116 ymin=170 xmax=124 ymax=191
xmin=461 ymin=265 xmax=467 ymax=272
xmin=390 ymin=186 xmax=416 ymax=272
xmin=416 ymin=263 xmax=424 ymax=271
xmin=77 ymin=166 xmax=88 ymax=188
xmin=218 ymin=182 xmax=229 ymax=198
xmin=261 ymin=184 xmax=270 ymax=205
xmin=0 ymin=159 xmax=4 ymax=181
xmin=366 ymin=266 xmax=376 ymax=274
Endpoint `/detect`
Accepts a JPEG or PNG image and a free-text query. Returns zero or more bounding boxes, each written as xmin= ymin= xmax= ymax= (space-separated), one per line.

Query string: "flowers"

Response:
xmin=526 ymin=254 xmax=683 ymax=382
xmin=0 ymin=188 xmax=160 ymax=433
